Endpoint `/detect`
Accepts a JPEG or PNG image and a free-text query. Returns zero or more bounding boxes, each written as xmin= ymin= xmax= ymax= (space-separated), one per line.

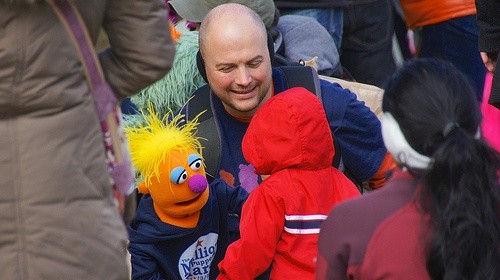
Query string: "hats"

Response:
xmin=168 ymin=0 xmax=275 ymax=30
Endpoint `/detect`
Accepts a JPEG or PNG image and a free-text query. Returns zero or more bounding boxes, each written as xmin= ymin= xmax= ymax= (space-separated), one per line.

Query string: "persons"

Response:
xmin=216 ymin=86 xmax=362 ymax=280
xmin=0 ymin=0 xmax=176 ymax=280
xmin=474 ymin=1 xmax=500 ymax=110
xmin=318 ymin=57 xmax=500 ymax=280
xmin=399 ymin=0 xmax=488 ymax=102
xmin=166 ymin=3 xmax=400 ymax=198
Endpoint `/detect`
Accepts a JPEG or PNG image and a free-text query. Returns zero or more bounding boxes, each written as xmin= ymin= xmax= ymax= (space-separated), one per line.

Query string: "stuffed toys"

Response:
xmin=123 ymin=108 xmax=249 ymax=280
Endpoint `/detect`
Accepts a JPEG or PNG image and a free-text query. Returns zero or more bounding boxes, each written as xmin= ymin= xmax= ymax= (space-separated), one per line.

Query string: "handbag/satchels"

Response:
xmin=93 ymin=87 xmax=135 ymax=196
